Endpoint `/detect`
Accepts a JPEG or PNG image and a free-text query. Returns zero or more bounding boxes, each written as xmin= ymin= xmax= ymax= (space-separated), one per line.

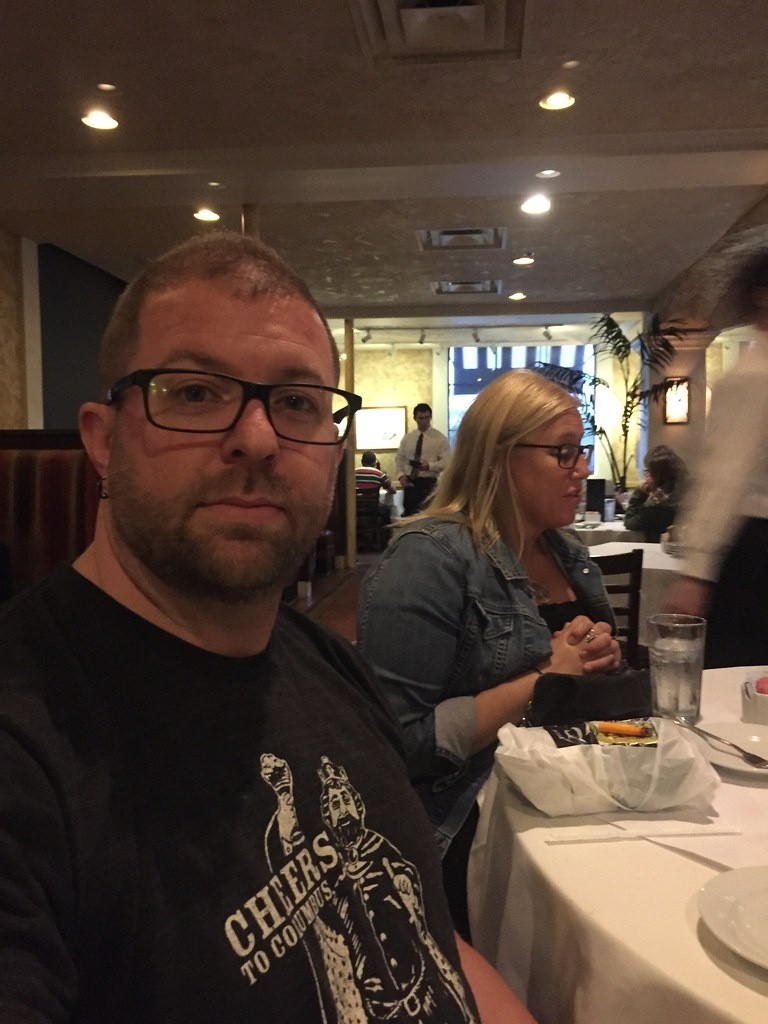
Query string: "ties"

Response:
xmin=408 ymin=433 xmax=424 ymax=482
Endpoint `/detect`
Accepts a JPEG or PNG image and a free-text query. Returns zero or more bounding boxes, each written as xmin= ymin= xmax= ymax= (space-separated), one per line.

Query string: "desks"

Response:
xmin=584 ymin=541 xmax=704 ymax=643
xmin=570 ymin=512 xmax=645 ymax=545
xmin=377 ymin=490 xmax=404 ymax=519
xmin=468 ymin=665 xmax=768 ymax=1024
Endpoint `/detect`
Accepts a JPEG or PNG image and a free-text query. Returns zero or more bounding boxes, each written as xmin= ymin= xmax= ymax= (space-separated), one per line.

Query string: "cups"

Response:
xmin=604 ymin=498 xmax=616 ymax=521
xmin=645 ymin=613 xmax=708 ymax=727
xmin=740 ymin=684 xmax=768 ymax=725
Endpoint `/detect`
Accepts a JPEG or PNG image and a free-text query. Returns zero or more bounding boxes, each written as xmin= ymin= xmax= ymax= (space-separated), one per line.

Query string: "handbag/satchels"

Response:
xmin=522 ymin=669 xmax=653 ymax=728
xmin=493 ymin=717 xmax=721 ymax=819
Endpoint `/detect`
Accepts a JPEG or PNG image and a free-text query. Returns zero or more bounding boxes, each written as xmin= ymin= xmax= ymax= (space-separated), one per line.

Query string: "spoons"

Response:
xmin=660 ymin=711 xmax=768 ymax=770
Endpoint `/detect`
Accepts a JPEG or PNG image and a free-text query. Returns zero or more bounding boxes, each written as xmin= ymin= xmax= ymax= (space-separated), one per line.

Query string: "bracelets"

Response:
xmin=533 ymin=666 xmax=544 ymax=676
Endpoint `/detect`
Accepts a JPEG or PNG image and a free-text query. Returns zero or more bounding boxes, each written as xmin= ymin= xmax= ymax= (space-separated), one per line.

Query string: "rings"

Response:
xmin=584 ymin=628 xmax=598 ymax=643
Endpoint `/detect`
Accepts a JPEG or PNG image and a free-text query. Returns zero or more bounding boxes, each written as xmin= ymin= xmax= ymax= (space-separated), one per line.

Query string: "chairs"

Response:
xmin=585 ymin=550 xmax=645 ymax=668
xmin=354 ymin=486 xmax=391 ymax=554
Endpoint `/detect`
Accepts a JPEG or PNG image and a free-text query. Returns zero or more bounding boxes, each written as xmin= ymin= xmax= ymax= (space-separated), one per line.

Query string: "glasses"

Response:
xmin=105 ymin=368 xmax=362 ymax=445
xmin=506 ymin=443 xmax=595 ymax=469
xmin=643 ymin=470 xmax=650 ymax=477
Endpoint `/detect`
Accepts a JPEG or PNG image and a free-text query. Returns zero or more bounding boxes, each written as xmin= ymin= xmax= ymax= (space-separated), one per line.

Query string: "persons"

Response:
xmin=624 ymin=445 xmax=689 ymax=542
xmin=356 ymin=404 xmax=451 ymax=556
xmin=0 ymin=233 xmax=540 ymax=1024
xmin=348 ymin=368 xmax=623 ymax=949
xmin=663 ymin=241 xmax=768 ymax=669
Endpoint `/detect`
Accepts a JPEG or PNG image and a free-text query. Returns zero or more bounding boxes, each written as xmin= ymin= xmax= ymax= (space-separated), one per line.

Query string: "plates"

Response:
xmin=696 ymin=721 xmax=768 ymax=775
xmin=697 ymin=863 xmax=768 ymax=971
